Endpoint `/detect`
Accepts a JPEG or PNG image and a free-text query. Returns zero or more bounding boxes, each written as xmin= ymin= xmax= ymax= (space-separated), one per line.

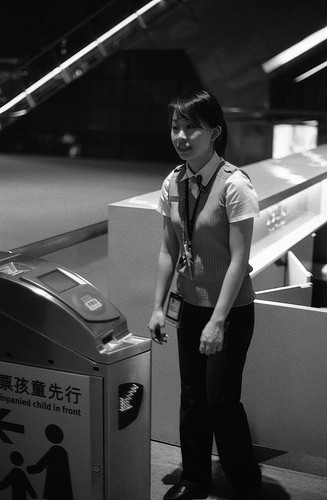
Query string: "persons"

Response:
xmin=146 ymin=87 xmax=262 ymax=500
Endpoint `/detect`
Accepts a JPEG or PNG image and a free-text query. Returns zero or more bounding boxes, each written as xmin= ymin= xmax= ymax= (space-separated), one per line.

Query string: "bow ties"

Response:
xmin=189 ymin=175 xmax=207 ymax=193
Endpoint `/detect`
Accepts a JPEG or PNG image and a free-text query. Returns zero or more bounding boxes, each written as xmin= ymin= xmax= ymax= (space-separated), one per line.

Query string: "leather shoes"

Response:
xmin=237 ymin=492 xmax=261 ymax=500
xmin=162 ymin=477 xmax=209 ymax=500
